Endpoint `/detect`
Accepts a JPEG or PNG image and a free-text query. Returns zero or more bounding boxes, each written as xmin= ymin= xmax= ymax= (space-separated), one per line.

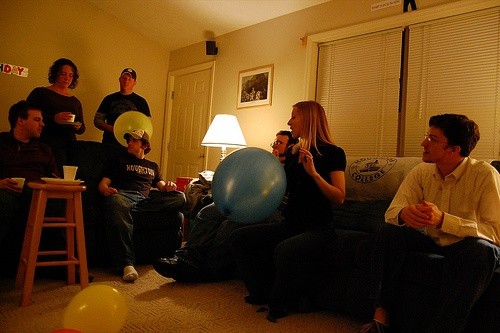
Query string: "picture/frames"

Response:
xmin=236 ymin=64 xmax=275 ymax=109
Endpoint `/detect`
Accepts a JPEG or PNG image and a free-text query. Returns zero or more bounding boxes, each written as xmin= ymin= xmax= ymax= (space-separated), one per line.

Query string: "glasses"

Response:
xmin=425 ymin=135 xmax=451 ymax=144
xmin=270 ymin=141 xmax=288 ymax=146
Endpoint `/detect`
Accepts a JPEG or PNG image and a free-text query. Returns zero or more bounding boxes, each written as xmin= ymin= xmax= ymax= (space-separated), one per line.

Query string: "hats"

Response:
xmin=124 ymin=128 xmax=149 ymax=141
xmin=121 ymin=68 xmax=136 ymax=79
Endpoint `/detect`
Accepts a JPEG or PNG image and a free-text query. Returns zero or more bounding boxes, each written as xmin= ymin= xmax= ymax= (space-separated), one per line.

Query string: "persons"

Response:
xmin=243 ymin=100 xmax=347 ymax=322
xmin=93 ymin=68 xmax=151 ymax=152
xmin=0 ymin=99 xmax=94 ymax=286
xmin=359 ymin=113 xmax=500 ymax=333
xmin=98 ymin=128 xmax=187 ymax=281
xmin=27 ymin=58 xmax=86 ymax=139
xmin=150 ymin=130 xmax=299 ymax=286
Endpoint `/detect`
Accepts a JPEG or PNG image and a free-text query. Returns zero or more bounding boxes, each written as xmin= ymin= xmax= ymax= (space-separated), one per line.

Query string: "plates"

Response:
xmin=41 ymin=178 xmax=84 ymax=185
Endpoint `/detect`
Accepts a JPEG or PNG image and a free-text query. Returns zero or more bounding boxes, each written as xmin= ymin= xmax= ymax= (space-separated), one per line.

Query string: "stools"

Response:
xmin=16 ymin=182 xmax=90 ymax=306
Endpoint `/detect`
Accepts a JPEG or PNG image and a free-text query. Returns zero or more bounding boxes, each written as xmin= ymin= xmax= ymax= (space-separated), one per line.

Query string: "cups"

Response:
xmin=67 ymin=115 xmax=75 ymax=122
xmin=62 ymin=165 xmax=78 ymax=181
xmin=11 ymin=178 xmax=25 ymax=192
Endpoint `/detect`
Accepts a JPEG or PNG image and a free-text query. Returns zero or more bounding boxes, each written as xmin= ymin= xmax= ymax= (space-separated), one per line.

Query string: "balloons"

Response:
xmin=64 ymin=284 xmax=128 ymax=333
xmin=114 ymin=111 xmax=153 ymax=148
xmin=211 ymin=147 xmax=287 ymax=225
xmin=50 ymin=329 xmax=86 ymax=333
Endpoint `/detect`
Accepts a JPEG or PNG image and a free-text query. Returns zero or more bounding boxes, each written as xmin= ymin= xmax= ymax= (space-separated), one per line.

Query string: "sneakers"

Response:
xmin=153 ymin=258 xmax=194 ymax=284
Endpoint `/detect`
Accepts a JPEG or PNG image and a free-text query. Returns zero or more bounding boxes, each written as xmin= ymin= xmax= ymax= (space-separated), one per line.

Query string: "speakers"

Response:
xmin=206 ymin=41 xmax=215 ymax=55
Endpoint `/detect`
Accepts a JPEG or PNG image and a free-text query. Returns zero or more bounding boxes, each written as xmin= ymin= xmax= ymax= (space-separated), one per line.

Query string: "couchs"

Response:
xmin=299 ymin=155 xmax=500 ymax=333
xmin=0 ymin=140 xmax=184 ymax=279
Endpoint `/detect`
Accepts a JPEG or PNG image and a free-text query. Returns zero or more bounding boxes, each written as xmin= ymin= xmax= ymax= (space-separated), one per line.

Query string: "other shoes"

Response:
xmin=75 ymin=268 xmax=94 ymax=284
xmin=245 ymin=293 xmax=288 ymax=319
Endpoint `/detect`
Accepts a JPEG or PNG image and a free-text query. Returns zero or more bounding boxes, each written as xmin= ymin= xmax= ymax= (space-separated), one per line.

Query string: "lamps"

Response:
xmin=200 ymin=114 xmax=247 ymax=162
